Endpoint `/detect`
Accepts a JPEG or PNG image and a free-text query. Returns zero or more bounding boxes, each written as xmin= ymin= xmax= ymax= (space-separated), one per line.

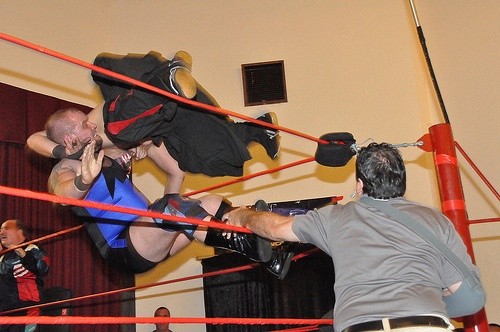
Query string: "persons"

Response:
xmin=150 ymin=307 xmax=174 ymax=332
xmin=0 ymin=219 xmax=49 ymax=332
xmin=26 ymin=50 xmax=280 ymax=177
xmin=46 ymin=106 xmax=297 ymax=281
xmin=222 ymin=141 xmax=486 ymax=332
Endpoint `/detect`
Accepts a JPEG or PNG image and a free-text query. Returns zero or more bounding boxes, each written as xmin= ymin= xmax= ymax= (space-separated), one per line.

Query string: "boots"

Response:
xmin=146 ymin=51 xmax=196 ymax=99
xmin=214 ymin=196 xmax=298 ymax=279
xmin=236 ymin=112 xmax=280 ymax=159
xmin=204 ymin=200 xmax=273 ymax=261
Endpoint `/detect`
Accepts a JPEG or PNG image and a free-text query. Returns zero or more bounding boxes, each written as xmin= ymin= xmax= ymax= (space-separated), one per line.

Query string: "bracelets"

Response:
xmin=74 ymin=175 xmax=91 ymax=192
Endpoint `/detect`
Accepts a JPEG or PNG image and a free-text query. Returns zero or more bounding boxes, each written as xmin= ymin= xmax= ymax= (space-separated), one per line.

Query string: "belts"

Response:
xmin=342 ymin=316 xmax=449 ymax=332
